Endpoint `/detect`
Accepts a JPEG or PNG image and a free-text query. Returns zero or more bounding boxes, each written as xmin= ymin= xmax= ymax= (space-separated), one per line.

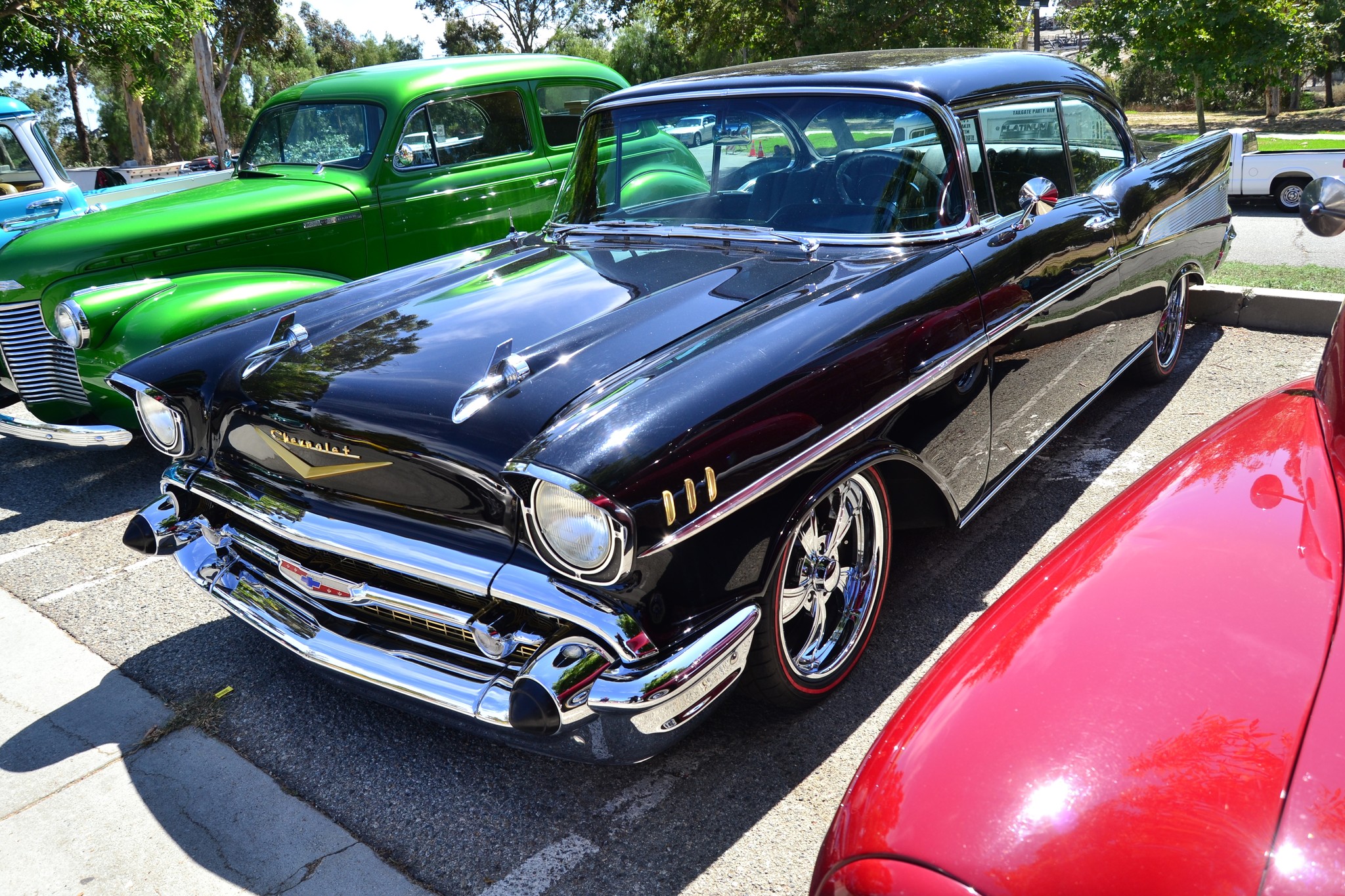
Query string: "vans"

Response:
xmin=890 ymin=100 xmax=1105 ymax=146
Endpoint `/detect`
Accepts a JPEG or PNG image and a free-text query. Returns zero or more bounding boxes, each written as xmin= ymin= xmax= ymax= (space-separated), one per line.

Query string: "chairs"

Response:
xmin=822 ymin=146 xmax=920 ymax=209
xmin=975 ymin=147 xmax=1103 ymax=215
xmin=479 ymin=114 xmax=528 ymax=156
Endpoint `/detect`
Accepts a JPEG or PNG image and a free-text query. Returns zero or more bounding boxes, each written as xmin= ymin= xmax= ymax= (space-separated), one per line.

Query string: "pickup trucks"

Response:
xmin=0 ymin=95 xmax=234 ymax=251
xmin=1192 ymin=127 xmax=1345 ymax=213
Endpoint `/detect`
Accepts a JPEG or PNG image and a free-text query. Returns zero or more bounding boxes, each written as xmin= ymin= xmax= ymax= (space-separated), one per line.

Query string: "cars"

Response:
xmin=0 ymin=51 xmax=711 ymax=451
xmin=105 ymin=46 xmax=1236 ymax=769
xmin=805 ymin=298 xmax=1345 ymax=896
xmin=166 ymin=153 xmax=241 ymax=177
xmin=664 ymin=114 xmax=728 ymax=147
xmin=397 ymin=131 xmax=459 ymax=150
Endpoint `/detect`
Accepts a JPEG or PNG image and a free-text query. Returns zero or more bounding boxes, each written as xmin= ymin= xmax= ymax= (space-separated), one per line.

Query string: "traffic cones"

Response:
xmin=747 ymin=140 xmax=758 ymax=157
xmin=756 ymin=141 xmax=766 ymax=159
